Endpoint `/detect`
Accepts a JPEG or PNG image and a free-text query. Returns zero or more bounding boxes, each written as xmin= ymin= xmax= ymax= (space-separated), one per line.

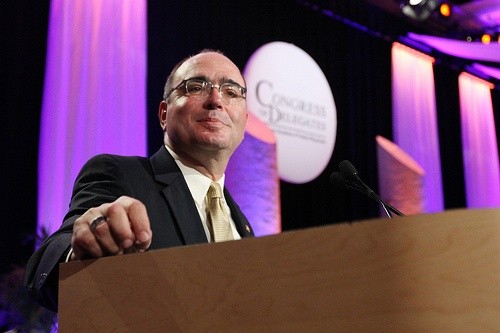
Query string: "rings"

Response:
xmin=89 ymin=216 xmax=107 ymax=231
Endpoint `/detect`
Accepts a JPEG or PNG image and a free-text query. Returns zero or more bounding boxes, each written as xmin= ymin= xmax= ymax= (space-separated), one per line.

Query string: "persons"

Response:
xmin=24 ymin=48 xmax=256 ymax=313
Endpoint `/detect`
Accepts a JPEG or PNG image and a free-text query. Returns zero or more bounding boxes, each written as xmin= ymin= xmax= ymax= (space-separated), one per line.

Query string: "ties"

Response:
xmin=207 ymin=182 xmax=236 ymax=242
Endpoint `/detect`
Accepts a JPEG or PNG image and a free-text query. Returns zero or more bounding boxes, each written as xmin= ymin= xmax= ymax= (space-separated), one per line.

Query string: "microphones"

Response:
xmin=330 ymin=171 xmax=407 ymax=217
xmin=339 ymin=160 xmax=393 ymax=219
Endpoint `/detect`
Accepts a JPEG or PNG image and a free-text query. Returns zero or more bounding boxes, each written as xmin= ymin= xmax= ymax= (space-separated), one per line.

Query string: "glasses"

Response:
xmin=166 ymin=77 xmax=247 ymax=103
